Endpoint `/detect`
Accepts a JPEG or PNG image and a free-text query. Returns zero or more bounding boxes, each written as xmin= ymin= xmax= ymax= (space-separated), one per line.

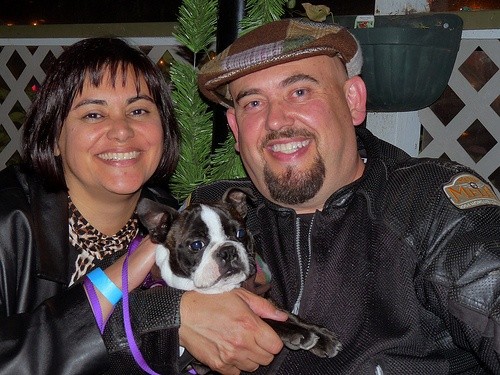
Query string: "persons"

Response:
xmin=103 ymin=19 xmax=500 ymax=375
xmin=0 ymin=36 xmax=182 ymax=375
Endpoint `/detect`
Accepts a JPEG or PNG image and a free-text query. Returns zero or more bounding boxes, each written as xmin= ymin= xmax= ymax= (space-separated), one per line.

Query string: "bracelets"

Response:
xmin=85 ymin=267 xmax=124 ymax=307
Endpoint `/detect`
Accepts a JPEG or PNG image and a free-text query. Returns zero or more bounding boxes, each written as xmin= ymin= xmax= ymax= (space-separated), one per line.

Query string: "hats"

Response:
xmin=198 ymin=18 xmax=363 ymax=109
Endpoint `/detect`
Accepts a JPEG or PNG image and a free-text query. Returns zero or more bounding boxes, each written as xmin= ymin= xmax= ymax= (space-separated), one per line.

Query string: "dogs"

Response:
xmin=136 ymin=186 xmax=343 ymax=375
